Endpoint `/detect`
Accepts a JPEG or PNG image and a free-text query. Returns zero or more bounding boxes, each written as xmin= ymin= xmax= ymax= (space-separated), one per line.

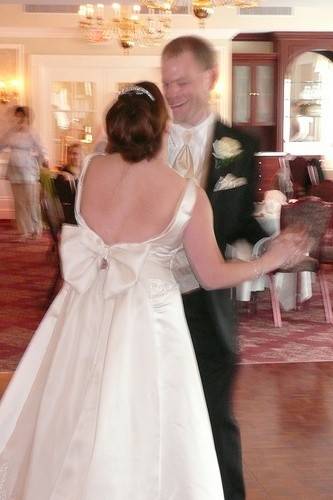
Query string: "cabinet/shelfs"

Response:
xmin=254 ymin=154 xmax=283 ymax=205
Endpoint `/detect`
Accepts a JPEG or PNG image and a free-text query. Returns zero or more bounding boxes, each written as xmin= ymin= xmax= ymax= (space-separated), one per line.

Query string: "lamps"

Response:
xmin=78 ymin=0 xmax=261 ymax=48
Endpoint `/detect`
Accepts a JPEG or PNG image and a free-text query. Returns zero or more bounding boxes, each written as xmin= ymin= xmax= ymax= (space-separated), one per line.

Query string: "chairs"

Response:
xmin=38 ymin=168 xmax=78 ymax=309
xmin=254 ymin=196 xmax=333 ymax=329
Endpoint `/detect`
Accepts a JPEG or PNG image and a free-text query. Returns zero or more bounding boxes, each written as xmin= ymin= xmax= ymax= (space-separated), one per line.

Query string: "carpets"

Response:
xmin=0 ymin=216 xmax=333 ymax=370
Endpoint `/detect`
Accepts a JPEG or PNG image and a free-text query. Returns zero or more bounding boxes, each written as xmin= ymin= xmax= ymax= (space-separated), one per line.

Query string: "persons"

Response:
xmin=0 ymin=106 xmax=49 ymax=239
xmin=56 ymin=144 xmax=82 ymax=225
xmin=0 ymin=81 xmax=306 ymax=499
xmin=164 ymin=36 xmax=306 ymax=500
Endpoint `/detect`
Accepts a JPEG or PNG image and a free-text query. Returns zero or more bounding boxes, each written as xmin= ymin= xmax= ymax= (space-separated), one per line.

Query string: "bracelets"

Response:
xmin=251 ymin=262 xmax=262 ymax=278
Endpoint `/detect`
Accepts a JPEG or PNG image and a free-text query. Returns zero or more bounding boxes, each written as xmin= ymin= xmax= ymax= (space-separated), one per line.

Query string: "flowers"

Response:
xmin=211 ymin=135 xmax=244 ymax=174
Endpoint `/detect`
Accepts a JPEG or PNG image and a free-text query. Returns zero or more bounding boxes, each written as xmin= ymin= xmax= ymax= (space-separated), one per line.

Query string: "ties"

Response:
xmin=172 ymin=130 xmax=194 ymax=179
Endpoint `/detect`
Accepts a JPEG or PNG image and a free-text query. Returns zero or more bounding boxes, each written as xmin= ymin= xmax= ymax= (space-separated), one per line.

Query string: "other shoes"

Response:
xmin=20 ymin=234 xmax=42 ymax=241
